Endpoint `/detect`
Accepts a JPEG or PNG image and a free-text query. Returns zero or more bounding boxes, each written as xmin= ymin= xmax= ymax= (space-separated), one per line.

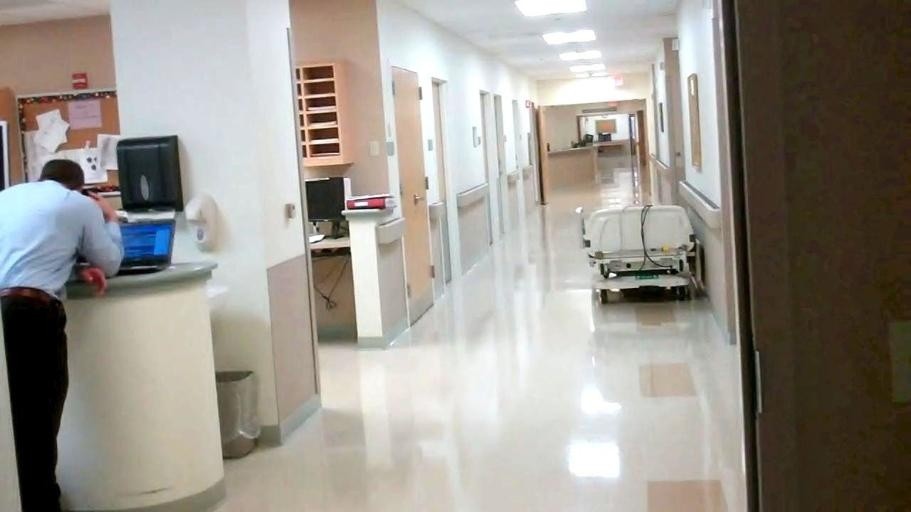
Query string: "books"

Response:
xmin=346 ymin=193 xmax=398 ymax=210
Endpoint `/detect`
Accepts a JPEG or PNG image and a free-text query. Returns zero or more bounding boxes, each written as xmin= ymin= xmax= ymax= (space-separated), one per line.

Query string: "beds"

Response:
xmin=575 ymin=204 xmax=703 ymax=303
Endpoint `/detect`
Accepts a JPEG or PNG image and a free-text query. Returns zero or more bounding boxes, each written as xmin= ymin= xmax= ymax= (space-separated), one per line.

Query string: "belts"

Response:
xmin=1 ymin=286 xmax=64 ymax=310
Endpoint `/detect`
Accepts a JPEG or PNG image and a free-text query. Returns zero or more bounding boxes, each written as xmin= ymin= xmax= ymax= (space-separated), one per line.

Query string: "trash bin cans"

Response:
xmin=215 ymin=371 xmax=256 ymax=458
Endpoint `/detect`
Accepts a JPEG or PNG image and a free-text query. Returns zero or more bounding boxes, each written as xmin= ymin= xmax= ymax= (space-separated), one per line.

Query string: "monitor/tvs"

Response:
xmin=117 ymin=135 xmax=184 ymax=211
xmin=304 ymin=177 xmax=345 ymax=239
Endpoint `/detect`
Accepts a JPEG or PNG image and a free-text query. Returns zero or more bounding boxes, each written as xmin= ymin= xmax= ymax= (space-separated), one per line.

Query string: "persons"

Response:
xmin=0 ymin=159 xmax=125 ymax=512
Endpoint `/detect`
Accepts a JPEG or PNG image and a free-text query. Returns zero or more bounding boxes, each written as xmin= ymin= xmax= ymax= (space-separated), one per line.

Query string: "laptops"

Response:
xmin=117 ymin=219 xmax=175 ymax=275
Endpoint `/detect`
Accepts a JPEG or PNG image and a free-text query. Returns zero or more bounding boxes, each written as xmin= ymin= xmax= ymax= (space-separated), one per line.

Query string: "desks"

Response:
xmin=309 ymin=233 xmax=349 ymax=258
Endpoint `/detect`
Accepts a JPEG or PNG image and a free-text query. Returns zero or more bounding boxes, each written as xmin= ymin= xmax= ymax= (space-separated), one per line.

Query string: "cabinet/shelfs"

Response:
xmin=294 ymin=62 xmax=355 ymax=167
